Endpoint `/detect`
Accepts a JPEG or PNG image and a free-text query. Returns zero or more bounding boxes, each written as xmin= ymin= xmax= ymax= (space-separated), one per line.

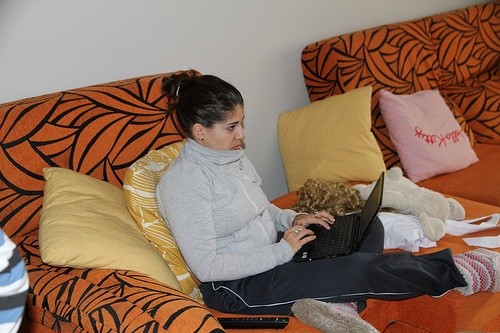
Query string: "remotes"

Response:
xmin=218 ymin=317 xmax=290 ymax=329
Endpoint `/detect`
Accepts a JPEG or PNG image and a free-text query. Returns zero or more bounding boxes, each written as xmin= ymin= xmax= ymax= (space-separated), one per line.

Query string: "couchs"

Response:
xmin=301 ymin=0 xmax=500 ymax=207
xmin=0 ymin=69 xmax=500 ymax=333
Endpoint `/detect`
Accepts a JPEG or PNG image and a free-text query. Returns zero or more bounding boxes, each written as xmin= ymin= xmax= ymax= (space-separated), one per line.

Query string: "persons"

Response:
xmin=155 ymin=69 xmax=500 ymax=333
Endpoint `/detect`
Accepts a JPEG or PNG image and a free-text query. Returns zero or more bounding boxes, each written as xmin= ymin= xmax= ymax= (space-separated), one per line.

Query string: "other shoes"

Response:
xmin=452 ymin=248 xmax=500 ymax=296
xmin=292 ymin=298 xmax=380 ymax=333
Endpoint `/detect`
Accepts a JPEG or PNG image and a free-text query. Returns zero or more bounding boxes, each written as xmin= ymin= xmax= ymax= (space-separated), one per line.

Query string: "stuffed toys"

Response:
xmin=351 ymin=166 xmax=466 ymax=242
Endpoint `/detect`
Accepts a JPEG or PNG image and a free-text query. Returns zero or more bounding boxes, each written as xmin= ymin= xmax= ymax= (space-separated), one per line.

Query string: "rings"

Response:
xmin=292 ymin=228 xmax=302 ymax=234
xmin=314 ymin=212 xmax=318 ymax=218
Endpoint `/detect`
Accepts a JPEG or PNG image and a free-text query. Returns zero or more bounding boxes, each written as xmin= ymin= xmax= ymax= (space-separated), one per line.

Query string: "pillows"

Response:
xmin=123 ymin=139 xmax=205 ymax=305
xmin=278 ymin=86 xmax=386 ymax=193
xmin=39 ymin=166 xmax=183 ymax=292
xmin=379 ymin=89 xmax=480 ymax=183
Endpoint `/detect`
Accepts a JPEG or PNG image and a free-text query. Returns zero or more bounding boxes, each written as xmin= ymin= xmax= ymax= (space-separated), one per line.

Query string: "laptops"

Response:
xmin=293 ymin=171 xmax=384 ymax=262
